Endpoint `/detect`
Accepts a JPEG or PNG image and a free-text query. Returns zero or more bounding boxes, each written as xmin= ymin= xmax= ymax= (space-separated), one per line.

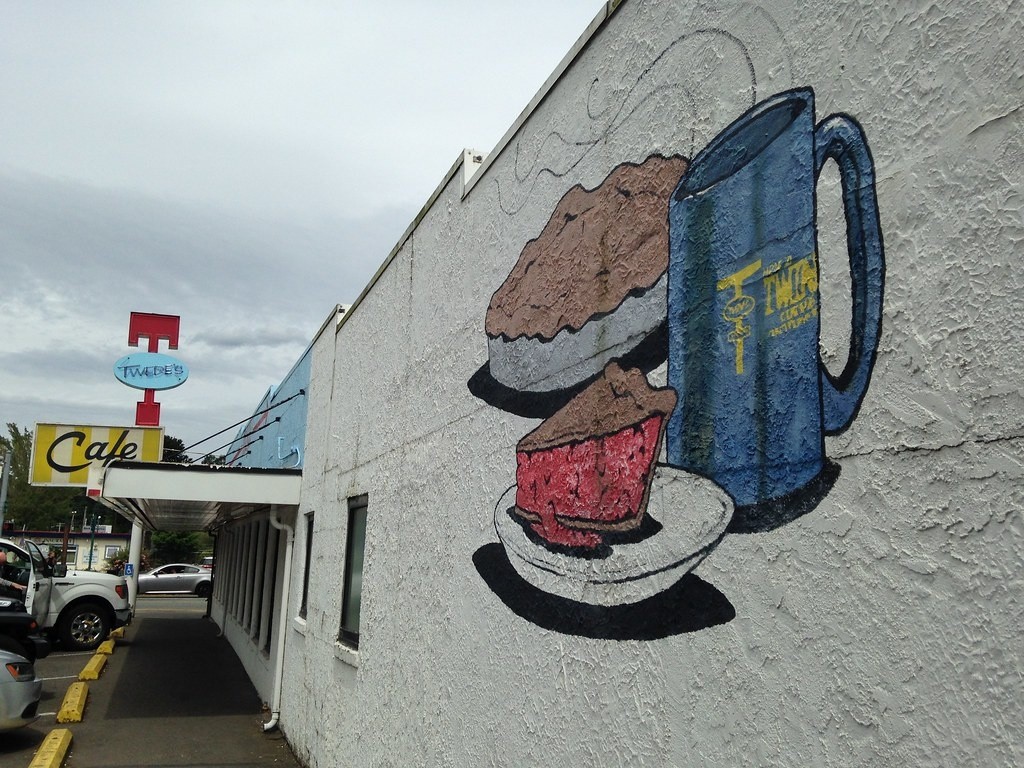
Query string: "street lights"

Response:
xmin=69 ymin=511 xmax=77 ymax=532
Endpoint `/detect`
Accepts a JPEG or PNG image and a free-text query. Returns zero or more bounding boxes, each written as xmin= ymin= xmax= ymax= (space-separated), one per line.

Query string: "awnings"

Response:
xmin=85 ymin=458 xmax=302 ymax=532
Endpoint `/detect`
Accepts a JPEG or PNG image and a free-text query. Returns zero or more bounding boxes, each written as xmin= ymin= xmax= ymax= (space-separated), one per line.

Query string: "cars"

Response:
xmin=0 ymin=649 xmax=42 ymax=734
xmin=0 ymin=596 xmax=53 ymax=666
xmin=0 ymin=538 xmax=132 ymax=651
xmin=120 ymin=563 xmax=214 ymax=598
xmin=195 ymin=557 xmax=213 ymax=573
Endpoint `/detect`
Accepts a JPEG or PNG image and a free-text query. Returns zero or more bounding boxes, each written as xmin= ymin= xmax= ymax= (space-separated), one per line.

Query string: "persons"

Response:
xmin=0 ymin=552 xmax=28 ymax=590
xmin=160 ymin=568 xmax=177 ymax=574
xmin=140 ymin=554 xmax=148 ymax=573
xmin=45 ymin=551 xmax=57 ymax=565
xmin=113 ymin=557 xmax=128 ymax=570
